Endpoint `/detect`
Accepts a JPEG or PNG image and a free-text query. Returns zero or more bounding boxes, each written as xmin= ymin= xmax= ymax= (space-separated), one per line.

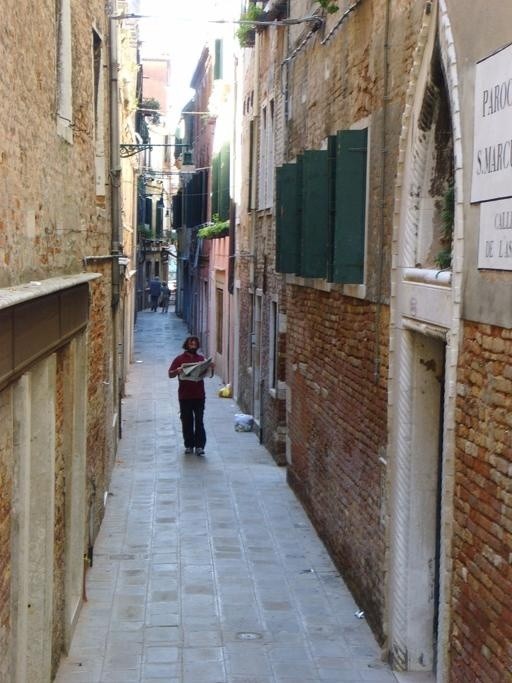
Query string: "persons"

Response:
xmin=149 ymin=275 xmax=161 ymax=311
xmin=168 ymin=336 xmax=215 ymax=456
xmin=160 ymin=280 xmax=170 ymax=312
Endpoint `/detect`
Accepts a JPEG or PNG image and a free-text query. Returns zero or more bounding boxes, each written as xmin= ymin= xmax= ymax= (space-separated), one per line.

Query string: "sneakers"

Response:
xmin=195 ymin=446 xmax=205 ymax=456
xmin=184 ymin=447 xmax=194 ymax=454
xmin=150 ymin=307 xmax=169 ymax=314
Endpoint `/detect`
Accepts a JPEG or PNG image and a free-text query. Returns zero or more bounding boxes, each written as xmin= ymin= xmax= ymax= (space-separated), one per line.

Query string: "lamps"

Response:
xmin=120 ymin=143 xmax=196 ymax=174
